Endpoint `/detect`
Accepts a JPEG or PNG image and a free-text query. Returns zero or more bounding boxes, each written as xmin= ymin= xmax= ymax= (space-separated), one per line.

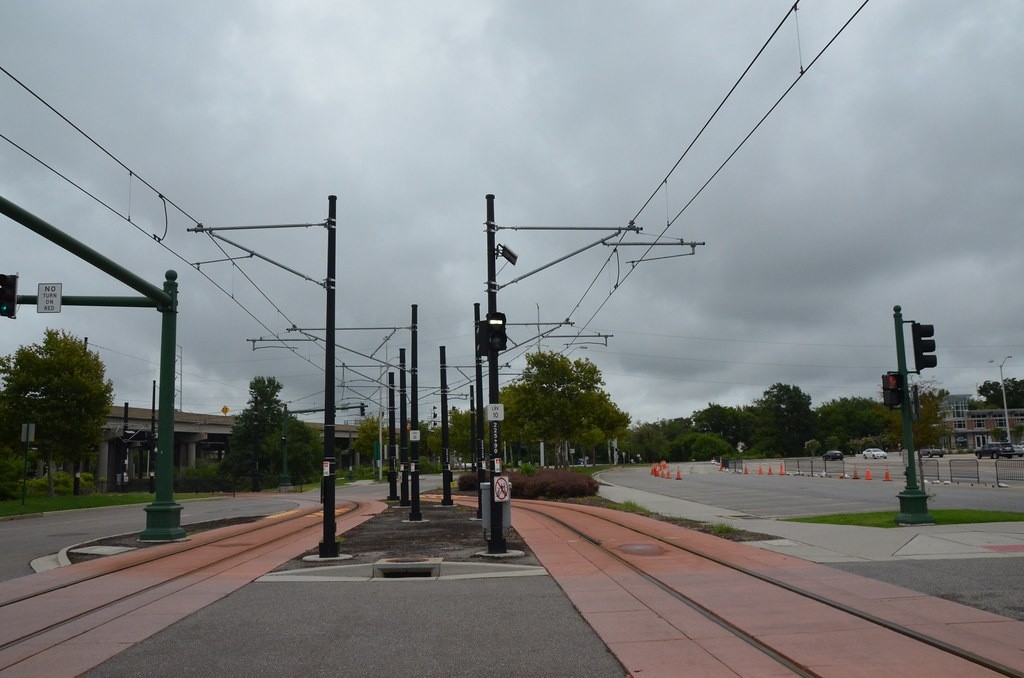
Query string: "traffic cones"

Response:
xmin=851 ymin=467 xmax=860 ymax=479
xmin=742 ymin=462 xmax=785 ymax=475
xmin=882 ymin=463 xmax=893 ymax=481
xmin=649 ymin=461 xmax=683 ymax=479
xmin=864 ymin=467 xmax=873 ymax=480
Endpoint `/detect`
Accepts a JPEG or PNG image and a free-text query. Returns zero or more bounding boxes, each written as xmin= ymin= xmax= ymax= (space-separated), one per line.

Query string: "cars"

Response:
xmin=822 ymin=450 xmax=844 ymax=461
xmin=862 ymin=448 xmax=887 ymax=460
xmin=921 ymin=444 xmax=945 ymax=458
xmin=1013 ymin=445 xmax=1024 ymax=457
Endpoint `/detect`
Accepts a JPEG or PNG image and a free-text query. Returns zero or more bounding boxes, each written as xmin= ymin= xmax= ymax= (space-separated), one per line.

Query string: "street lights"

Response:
xmin=987 ymin=355 xmax=1013 ymax=443
xmin=564 ymin=345 xmax=588 ymax=462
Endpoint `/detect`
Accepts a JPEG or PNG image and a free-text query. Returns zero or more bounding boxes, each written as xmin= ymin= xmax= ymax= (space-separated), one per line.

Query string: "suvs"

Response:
xmin=974 ymin=441 xmax=1015 ymax=460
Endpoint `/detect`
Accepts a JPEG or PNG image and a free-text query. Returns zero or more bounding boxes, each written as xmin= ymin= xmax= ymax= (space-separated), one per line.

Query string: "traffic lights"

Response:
xmin=433 ymin=412 xmax=437 ymax=418
xmin=433 ymin=422 xmax=438 ymax=426
xmin=911 ymin=322 xmax=938 ymax=370
xmin=485 ymin=312 xmax=507 ymax=351
xmin=0 ymin=274 xmax=19 ymax=319
xmin=360 ymin=402 xmax=366 ymax=417
xmin=433 ymin=406 xmax=438 ymax=409
xmin=881 ymin=373 xmax=904 ymax=407
xmin=474 ymin=320 xmax=488 ymax=356
